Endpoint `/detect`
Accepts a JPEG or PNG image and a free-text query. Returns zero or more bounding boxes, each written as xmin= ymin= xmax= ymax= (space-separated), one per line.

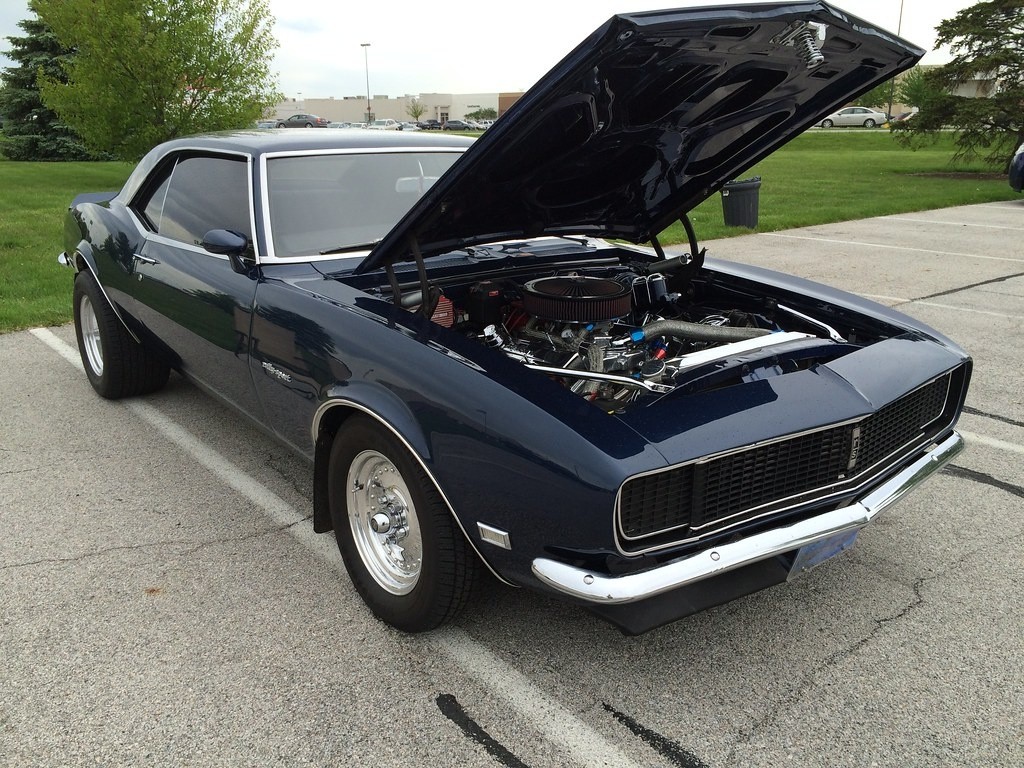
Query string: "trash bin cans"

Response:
xmin=720 ymin=176 xmax=761 ymax=228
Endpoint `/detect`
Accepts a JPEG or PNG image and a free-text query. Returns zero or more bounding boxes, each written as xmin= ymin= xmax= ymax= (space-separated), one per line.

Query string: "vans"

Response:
xmin=426 ymin=119 xmax=442 ymax=130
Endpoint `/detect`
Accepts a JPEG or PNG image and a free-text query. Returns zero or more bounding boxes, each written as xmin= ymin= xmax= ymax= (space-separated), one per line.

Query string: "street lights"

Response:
xmin=297 ymin=93 xmax=302 ymax=109
xmin=361 ymin=44 xmax=372 ymax=125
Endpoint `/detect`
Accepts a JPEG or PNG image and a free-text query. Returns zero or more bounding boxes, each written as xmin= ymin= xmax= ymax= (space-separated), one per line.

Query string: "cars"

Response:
xmin=442 ymin=120 xmax=475 ymax=131
xmin=348 ymin=122 xmax=367 ymax=129
xmin=464 ymin=120 xmax=496 ymax=131
xmin=401 ymin=124 xmax=422 ymax=132
xmin=813 ymin=106 xmax=887 ymax=129
xmin=416 ymin=121 xmax=431 ymax=130
xmin=277 ymin=114 xmax=328 ymax=128
xmin=1008 ymin=144 xmax=1024 ymax=194
xmin=258 ymin=122 xmax=278 ymax=128
xmin=890 ymin=112 xmax=916 ymax=126
xmin=368 ymin=119 xmax=403 ymax=129
xmin=56 ymin=0 xmax=974 ymax=633
xmin=327 ymin=122 xmax=346 ymax=129
